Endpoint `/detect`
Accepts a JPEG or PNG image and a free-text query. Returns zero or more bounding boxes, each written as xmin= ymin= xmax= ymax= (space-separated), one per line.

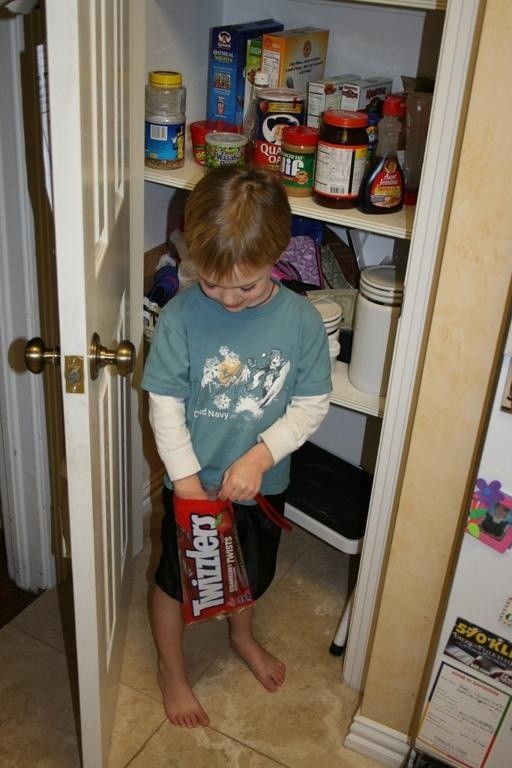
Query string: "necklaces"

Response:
xmin=246 ymin=282 xmax=277 ymax=311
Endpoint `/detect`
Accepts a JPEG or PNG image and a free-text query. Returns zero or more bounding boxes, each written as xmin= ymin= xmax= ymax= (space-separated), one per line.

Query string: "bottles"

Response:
xmin=357 ymin=95 xmax=406 ymax=215
xmin=143 ymin=69 xmax=188 ymax=171
xmin=242 ymin=71 xmax=270 ymax=170
xmin=348 ymin=264 xmax=407 ymax=397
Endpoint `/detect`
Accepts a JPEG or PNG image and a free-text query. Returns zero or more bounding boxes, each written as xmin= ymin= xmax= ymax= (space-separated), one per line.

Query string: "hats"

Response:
xmin=267 ymin=117 xmax=297 ymax=131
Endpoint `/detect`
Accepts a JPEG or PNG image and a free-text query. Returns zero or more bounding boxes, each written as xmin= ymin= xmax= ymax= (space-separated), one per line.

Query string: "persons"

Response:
xmin=481 ymin=502 xmax=512 ymax=541
xmin=138 ymin=160 xmax=336 ymax=730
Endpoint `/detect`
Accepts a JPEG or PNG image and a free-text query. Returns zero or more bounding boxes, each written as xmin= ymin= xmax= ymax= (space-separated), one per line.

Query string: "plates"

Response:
xmin=307 ymin=300 xmax=343 ymax=334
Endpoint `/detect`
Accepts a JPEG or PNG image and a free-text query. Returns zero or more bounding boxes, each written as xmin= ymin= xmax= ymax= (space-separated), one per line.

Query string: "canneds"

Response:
xmin=245 ymin=89 xmax=305 ymax=180
xmin=278 ymin=124 xmax=319 ymax=198
xmin=313 ymin=108 xmax=368 ymax=208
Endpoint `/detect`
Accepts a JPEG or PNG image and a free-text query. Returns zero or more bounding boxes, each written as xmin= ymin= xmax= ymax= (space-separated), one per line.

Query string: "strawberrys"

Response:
xmin=215 ymin=510 xmax=232 ymax=533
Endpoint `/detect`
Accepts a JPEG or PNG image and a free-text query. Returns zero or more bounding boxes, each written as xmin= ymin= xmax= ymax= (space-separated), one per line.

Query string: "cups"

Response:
xmin=328 ymin=328 xmax=342 ymax=374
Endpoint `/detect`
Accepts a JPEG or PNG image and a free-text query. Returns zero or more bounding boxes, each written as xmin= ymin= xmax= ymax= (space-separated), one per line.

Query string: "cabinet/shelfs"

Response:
xmin=127 ymin=0 xmax=512 ymax=690
